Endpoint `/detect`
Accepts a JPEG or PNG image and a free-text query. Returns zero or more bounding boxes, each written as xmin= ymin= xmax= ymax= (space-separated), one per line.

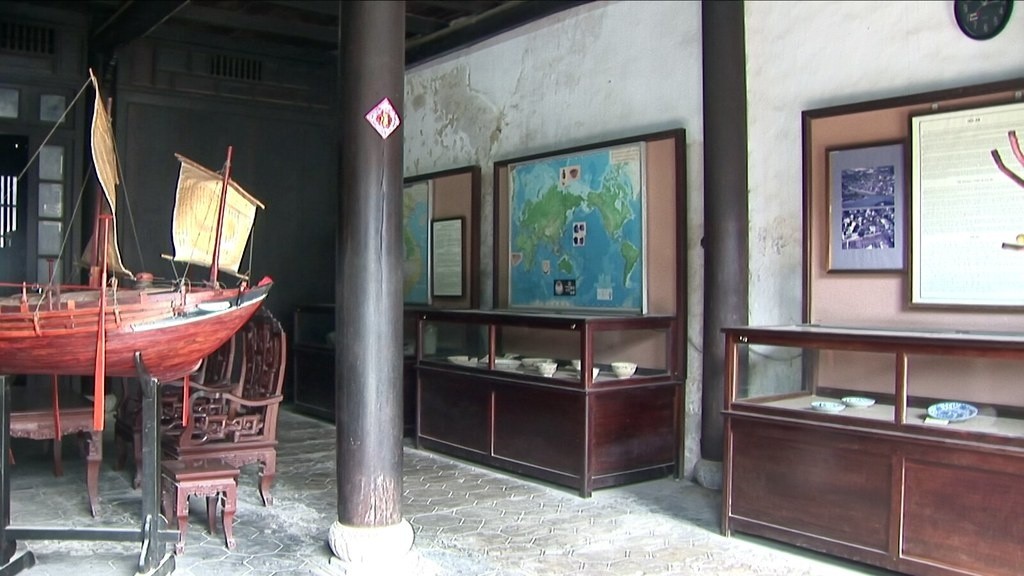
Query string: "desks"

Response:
xmin=10 ymin=384 xmax=101 ymax=520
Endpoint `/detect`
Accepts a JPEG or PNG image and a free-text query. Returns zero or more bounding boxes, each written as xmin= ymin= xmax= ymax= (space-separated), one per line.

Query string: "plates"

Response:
xmin=809 ymin=401 xmax=845 ymax=413
xmin=927 ymin=402 xmax=979 ymax=423
xmin=841 ymin=397 xmax=875 ymax=409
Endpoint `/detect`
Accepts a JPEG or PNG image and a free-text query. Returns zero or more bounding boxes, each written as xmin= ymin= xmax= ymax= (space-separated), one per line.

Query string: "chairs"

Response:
xmin=113 ymin=332 xmax=238 ymax=486
xmin=159 ymin=304 xmax=288 ymax=506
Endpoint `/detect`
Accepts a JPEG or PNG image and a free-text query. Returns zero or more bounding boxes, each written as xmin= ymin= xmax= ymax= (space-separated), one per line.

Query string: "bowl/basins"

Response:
xmin=572 ymin=359 xmax=581 ymax=371
xmin=522 ymin=358 xmax=552 ymax=370
xmin=577 ymin=366 xmax=600 ymax=379
xmin=495 ymin=360 xmax=521 ymax=369
xmin=537 ymin=362 xmax=558 ymax=377
xmin=611 ymin=362 xmax=638 ymax=379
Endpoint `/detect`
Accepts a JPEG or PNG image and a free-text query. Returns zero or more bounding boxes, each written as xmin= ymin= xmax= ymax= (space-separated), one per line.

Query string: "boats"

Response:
xmin=0 ymin=66 xmax=274 ymax=444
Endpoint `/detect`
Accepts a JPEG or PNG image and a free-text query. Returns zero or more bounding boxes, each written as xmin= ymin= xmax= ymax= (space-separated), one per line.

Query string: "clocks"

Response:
xmin=953 ymin=0 xmax=1015 ymax=40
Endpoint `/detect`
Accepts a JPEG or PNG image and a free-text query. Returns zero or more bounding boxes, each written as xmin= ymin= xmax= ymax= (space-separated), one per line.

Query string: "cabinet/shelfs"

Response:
xmin=111 ymin=41 xmax=340 ymax=327
xmin=293 ymin=304 xmax=429 ymax=420
xmin=414 ymin=309 xmax=679 ymax=498
xmin=719 ymin=321 xmax=1023 ymax=576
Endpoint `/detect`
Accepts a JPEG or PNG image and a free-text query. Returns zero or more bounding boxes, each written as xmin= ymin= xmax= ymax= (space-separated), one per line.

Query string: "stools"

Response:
xmin=161 ymin=456 xmax=240 ymax=555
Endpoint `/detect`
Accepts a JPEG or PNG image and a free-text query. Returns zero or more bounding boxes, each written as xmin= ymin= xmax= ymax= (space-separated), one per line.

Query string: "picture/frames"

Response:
xmin=826 ymin=141 xmax=909 ymax=273
